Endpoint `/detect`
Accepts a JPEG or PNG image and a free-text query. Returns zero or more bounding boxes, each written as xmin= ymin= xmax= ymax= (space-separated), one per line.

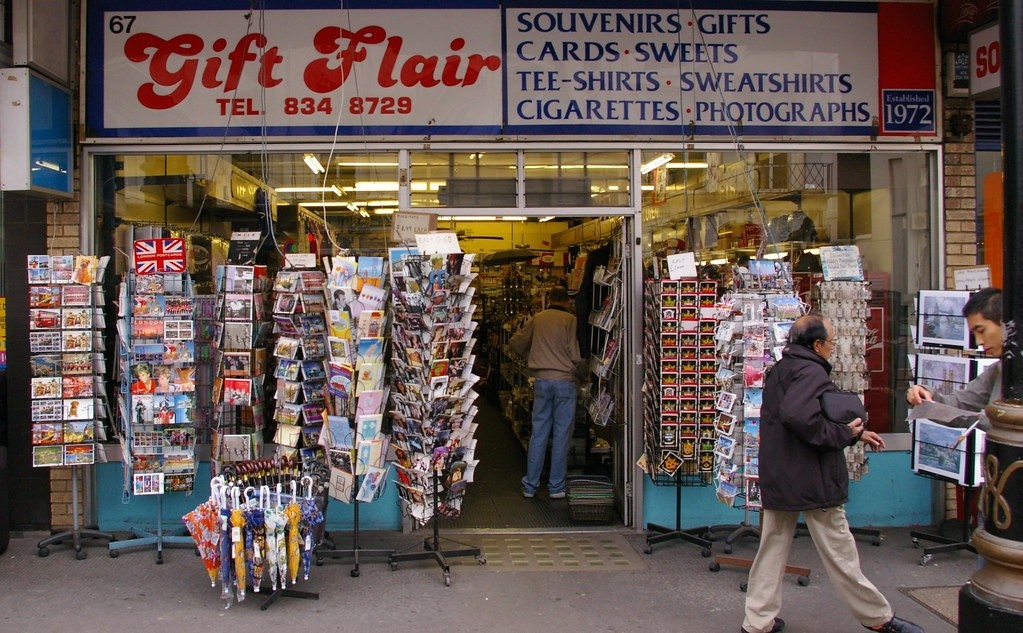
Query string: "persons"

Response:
xmin=740 ymin=314 xmax=925 ymax=632
xmin=331 ymin=288 xmax=353 ymax=319
xmin=156 ymin=406 xmax=174 ymax=423
xmin=154 ymin=365 xmax=175 ymax=392
xmin=904 ymin=287 xmax=1004 ymax=433
xmin=236 ymin=356 xmax=244 ymax=370
xmin=509 ymin=284 xmax=581 ymax=500
xmin=225 ymin=356 xmax=233 ymax=369
xmin=131 ymin=363 xmax=155 ymax=395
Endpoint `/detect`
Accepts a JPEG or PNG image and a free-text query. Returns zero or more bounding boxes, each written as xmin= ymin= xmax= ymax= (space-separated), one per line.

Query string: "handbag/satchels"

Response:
xmin=821 ymin=391 xmax=866 ymax=425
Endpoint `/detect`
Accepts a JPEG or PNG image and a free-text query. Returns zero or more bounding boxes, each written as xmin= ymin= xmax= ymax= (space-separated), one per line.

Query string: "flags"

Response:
xmin=134 ymin=239 xmax=186 ymax=272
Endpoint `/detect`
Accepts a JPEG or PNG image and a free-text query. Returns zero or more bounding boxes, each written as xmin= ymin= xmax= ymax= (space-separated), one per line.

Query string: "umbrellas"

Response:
xmin=482 ymin=248 xmax=541 ymax=266
xmin=182 ymin=455 xmax=325 ymax=595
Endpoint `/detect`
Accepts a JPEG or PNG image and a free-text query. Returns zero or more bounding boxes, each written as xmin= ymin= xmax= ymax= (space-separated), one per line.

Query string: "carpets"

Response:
xmin=440 ymin=531 xmax=645 ymax=573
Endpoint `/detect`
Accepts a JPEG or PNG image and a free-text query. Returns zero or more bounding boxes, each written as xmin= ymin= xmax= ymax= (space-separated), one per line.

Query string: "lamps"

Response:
xmin=302 ymin=153 xmax=372 ymax=219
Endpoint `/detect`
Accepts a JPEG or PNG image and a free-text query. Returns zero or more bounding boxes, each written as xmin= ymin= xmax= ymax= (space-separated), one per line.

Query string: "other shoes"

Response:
xmin=522 ymin=490 xmax=534 ymax=497
xmin=549 ymin=492 xmax=565 ymax=499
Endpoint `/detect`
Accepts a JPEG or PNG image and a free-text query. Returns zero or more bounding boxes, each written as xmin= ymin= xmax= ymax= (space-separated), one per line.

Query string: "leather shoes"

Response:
xmin=861 ymin=614 xmax=925 ymax=633
xmin=741 ymin=617 xmax=785 ymax=633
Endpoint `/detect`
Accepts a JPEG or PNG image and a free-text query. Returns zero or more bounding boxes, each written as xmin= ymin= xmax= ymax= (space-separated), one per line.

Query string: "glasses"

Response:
xmin=822 ymin=338 xmax=839 ymax=344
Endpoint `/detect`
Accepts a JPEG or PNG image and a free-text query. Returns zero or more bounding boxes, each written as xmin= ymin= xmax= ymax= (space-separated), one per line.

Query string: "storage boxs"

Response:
xmin=563 ymin=475 xmax=621 ymax=526
